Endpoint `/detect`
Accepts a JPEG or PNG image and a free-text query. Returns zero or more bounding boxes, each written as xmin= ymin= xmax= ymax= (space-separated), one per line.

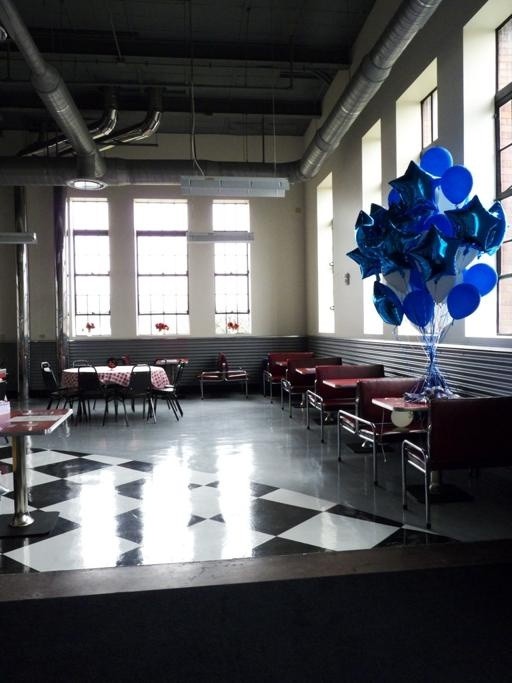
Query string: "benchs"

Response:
xmin=197 ymin=352 xmax=249 ymax=400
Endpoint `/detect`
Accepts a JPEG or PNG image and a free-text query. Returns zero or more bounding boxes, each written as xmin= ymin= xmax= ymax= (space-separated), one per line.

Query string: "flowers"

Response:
xmin=86 ymin=323 xmax=94 ymax=332
xmin=228 ymin=322 xmax=239 ymax=330
xmin=155 ymin=323 xmax=169 ymax=332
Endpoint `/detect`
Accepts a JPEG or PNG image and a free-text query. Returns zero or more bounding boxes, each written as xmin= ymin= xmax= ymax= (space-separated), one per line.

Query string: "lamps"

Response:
xmin=181 ymin=53 xmax=290 ymax=198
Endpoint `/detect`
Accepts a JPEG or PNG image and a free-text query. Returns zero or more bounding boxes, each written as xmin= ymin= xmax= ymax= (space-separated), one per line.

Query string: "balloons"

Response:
xmin=344 ymin=144 xmax=508 ymax=328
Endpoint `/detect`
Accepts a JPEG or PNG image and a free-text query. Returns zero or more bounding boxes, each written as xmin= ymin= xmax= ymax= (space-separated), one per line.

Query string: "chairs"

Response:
xmin=40 ymin=357 xmax=185 ymax=427
xmin=121 ymin=354 xmax=130 ymax=364
